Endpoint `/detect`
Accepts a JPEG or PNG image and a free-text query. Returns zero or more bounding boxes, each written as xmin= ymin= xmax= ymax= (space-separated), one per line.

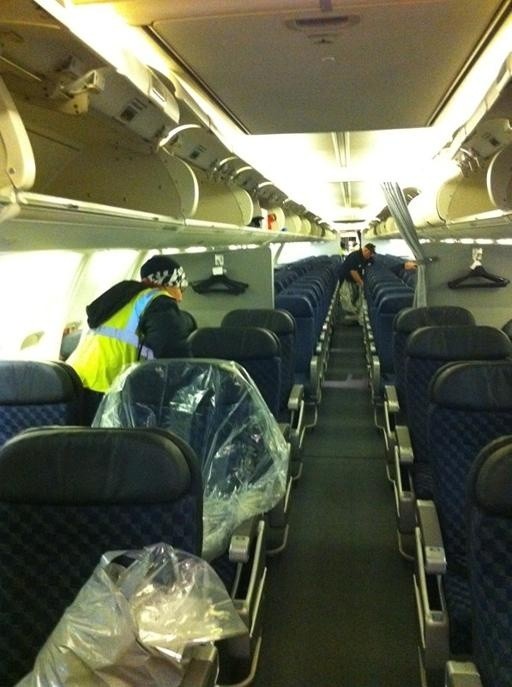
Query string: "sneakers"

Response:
xmin=342 ymin=320 xmax=364 ymax=329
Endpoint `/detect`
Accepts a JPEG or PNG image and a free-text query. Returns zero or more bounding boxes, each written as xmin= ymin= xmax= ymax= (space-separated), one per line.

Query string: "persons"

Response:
xmin=340 ymin=243 xmax=377 ymax=320
xmin=63 ymin=253 xmax=186 ymax=426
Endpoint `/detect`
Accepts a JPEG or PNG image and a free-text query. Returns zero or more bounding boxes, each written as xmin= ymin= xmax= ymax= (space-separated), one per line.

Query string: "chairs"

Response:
xmin=1 ymin=255 xmax=344 ymax=687
xmin=361 ymin=252 xmax=511 ymax=687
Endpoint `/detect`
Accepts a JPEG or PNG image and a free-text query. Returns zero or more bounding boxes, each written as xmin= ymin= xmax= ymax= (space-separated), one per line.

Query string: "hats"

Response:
xmin=365 ymin=243 xmax=377 ymax=254
xmin=140 ymin=255 xmax=189 ymax=289
